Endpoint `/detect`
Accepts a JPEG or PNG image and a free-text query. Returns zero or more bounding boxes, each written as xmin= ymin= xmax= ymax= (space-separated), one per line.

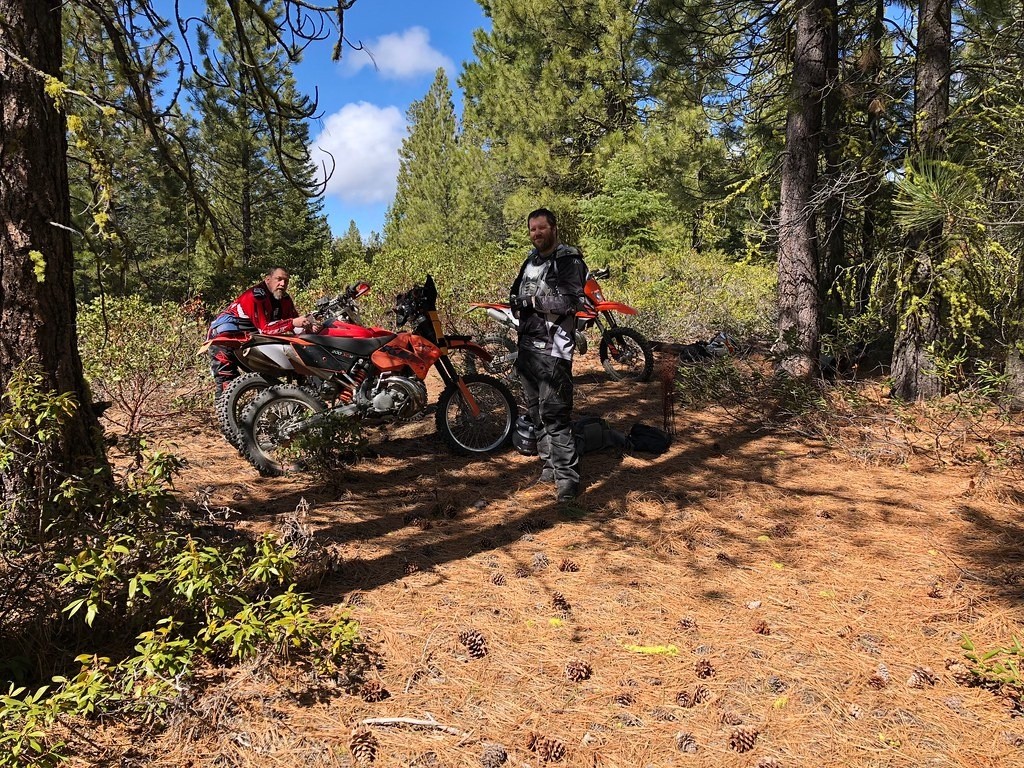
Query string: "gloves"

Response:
xmin=508 ymin=295 xmax=533 ymax=313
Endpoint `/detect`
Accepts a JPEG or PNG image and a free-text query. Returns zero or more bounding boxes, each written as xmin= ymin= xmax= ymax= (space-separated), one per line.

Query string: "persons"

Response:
xmin=509 ymin=208 xmax=585 ymax=502
xmin=206 ymin=266 xmax=321 ymax=407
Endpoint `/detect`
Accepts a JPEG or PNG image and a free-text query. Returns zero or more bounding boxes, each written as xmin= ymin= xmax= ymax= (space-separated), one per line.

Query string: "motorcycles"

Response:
xmin=236 ymin=273 xmax=519 ymax=477
xmin=464 ymin=265 xmax=654 ymax=386
xmin=196 ymin=281 xmax=428 ymax=450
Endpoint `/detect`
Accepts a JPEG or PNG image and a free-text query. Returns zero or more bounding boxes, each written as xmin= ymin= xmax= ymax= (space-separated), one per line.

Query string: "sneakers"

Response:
xmin=555 ymin=480 xmax=576 ymax=503
xmin=540 ymin=468 xmax=554 ymax=481
